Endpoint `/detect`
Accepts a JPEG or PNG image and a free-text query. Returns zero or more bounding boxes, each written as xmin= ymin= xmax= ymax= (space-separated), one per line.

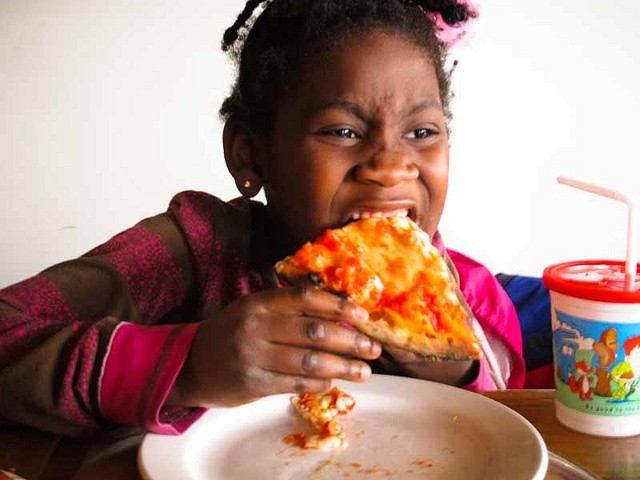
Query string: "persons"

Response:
xmin=0 ymin=0 xmax=527 ymax=435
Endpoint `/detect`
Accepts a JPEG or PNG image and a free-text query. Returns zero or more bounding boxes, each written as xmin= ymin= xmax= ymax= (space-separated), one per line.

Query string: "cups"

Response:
xmin=542 ymin=259 xmax=640 ymax=438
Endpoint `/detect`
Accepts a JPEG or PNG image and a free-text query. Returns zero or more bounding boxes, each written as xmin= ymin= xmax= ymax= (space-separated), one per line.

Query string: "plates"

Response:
xmin=135 ymin=372 xmax=549 ymax=480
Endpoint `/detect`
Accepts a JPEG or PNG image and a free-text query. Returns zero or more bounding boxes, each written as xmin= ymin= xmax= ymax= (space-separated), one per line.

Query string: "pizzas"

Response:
xmin=274 ymin=214 xmax=485 ymax=364
xmin=282 ymin=385 xmax=357 ymax=452
